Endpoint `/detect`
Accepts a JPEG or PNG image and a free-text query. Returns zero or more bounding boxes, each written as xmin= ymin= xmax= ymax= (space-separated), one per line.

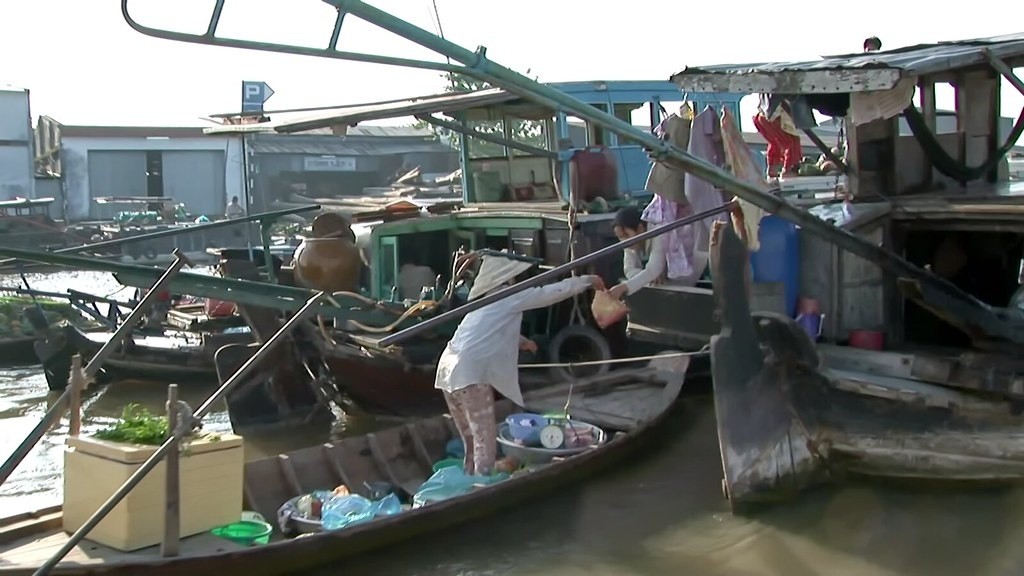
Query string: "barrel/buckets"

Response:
xmin=568 ymin=145 xmax=617 ymax=201
xmin=851 ymin=329 xmax=886 ymax=352
xmin=473 ymin=171 xmax=502 ymax=201
xmin=791 ymin=295 xmax=821 ymax=345
xmin=751 ymin=216 xmax=801 ymax=317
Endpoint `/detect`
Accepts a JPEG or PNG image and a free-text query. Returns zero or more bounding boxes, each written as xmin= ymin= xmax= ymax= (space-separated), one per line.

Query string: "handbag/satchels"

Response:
xmin=592 ymin=287 xmax=628 ymax=328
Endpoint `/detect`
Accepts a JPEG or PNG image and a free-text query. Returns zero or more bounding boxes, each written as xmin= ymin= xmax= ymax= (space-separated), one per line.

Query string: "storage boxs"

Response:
xmin=62 ymin=431 xmax=244 ymax=551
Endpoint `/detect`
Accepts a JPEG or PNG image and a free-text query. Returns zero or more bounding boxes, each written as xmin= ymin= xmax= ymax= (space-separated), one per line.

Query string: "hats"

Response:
xmin=467 ymin=249 xmax=542 ymax=301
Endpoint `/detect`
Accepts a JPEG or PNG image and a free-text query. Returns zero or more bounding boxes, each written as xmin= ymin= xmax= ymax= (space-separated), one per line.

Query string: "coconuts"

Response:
xmin=797 ymin=146 xmax=842 ymax=176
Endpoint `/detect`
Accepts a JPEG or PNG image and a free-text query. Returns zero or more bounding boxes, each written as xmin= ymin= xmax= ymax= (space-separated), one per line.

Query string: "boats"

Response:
xmin=0 ymin=0 xmax=1024 ymax=522
xmin=0 ymin=345 xmax=696 ymax=575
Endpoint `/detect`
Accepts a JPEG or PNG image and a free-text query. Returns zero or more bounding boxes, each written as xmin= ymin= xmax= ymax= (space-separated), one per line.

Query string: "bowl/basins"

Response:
xmin=213 ymin=520 xmax=273 ymax=547
xmin=281 ymin=493 xmax=375 ymax=534
xmin=492 ymin=415 xmax=607 ymax=468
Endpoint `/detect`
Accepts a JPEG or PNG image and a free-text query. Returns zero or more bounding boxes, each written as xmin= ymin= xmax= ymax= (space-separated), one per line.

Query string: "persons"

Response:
xmin=605 ymin=207 xmax=711 ymax=300
xmin=863 ymin=37 xmax=881 ymax=52
xmin=225 ymin=196 xmax=246 ymax=220
xmin=432 ymin=255 xmax=605 ymax=478
xmin=752 ymin=92 xmax=802 ymax=178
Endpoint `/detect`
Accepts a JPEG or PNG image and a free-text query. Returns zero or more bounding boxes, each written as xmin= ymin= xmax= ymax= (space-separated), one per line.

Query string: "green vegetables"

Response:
xmin=90 ymin=403 xmax=206 ymax=445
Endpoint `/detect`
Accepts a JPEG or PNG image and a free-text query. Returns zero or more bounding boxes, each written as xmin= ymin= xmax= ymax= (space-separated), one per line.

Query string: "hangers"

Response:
xmin=676 ymin=91 xmax=726 ymax=118
